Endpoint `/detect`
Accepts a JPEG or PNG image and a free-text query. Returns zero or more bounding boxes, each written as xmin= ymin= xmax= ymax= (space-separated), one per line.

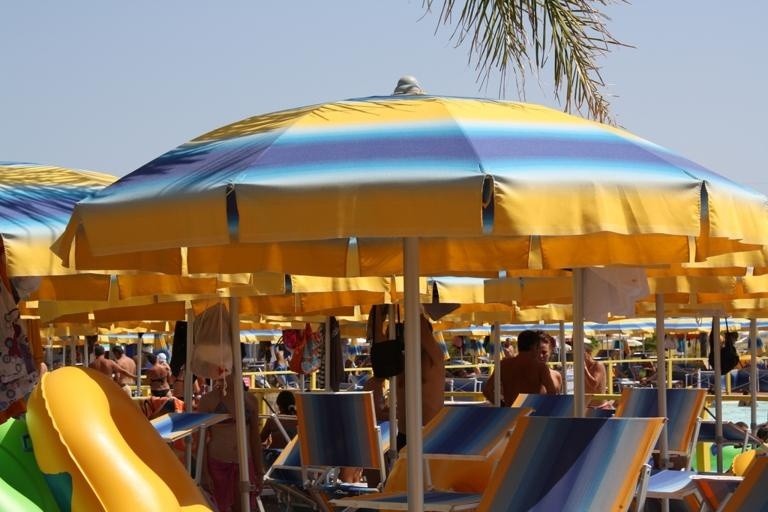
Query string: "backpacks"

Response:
xmin=191 ymin=303 xmax=235 ymax=379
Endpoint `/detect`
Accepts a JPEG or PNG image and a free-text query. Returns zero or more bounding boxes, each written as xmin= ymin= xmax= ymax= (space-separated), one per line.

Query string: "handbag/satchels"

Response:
xmin=709 ymin=317 xmax=739 ymax=374
xmin=290 ymin=323 xmax=322 ymax=374
xmin=371 ymin=340 xmax=405 ymax=377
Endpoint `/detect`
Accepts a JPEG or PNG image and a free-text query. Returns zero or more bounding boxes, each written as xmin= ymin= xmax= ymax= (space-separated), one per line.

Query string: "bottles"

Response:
xmin=566 ymin=364 xmax=574 ymax=396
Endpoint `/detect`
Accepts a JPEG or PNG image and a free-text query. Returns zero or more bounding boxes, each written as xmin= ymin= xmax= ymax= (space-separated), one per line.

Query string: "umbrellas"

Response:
xmin=47 ymin=74 xmax=768 ymax=510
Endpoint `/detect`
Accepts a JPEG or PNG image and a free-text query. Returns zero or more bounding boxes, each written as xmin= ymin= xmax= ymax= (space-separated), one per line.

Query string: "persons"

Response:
xmin=258 ymin=314 xmax=445 ymax=484
xmin=81 ymin=333 xmax=209 ymax=402
xmin=1 ymin=235 xmax=36 ymax=422
xmin=480 ymin=324 xmax=762 ymax=410
